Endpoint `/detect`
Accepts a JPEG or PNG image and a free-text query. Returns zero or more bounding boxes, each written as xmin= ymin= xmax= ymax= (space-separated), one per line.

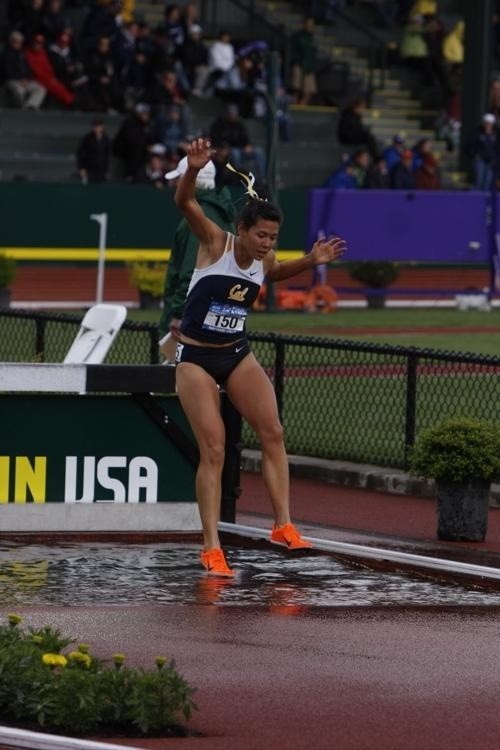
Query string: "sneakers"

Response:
xmin=200 ymin=548 xmax=236 ymax=578
xmin=270 ymin=522 xmax=313 ymax=550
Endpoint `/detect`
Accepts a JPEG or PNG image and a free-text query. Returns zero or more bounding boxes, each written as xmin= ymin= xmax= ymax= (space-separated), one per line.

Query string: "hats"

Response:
xmin=165 ymin=154 xmax=216 ymax=190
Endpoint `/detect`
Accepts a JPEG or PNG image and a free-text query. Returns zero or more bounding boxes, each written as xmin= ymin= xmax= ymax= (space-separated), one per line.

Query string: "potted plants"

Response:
xmin=412 ymin=414 xmax=499 ymax=545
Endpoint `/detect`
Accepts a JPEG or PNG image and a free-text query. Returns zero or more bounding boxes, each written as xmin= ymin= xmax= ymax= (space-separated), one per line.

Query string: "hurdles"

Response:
xmin=1 ymin=362 xmax=243 ymax=521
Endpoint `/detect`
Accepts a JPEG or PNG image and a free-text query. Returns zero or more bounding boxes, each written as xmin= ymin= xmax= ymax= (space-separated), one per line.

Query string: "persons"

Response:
xmin=176 ymin=138 xmax=347 ymax=577
xmin=162 ymin=121 xmax=269 ymax=363
xmin=1 ymin=1 xmax=500 ymax=189
